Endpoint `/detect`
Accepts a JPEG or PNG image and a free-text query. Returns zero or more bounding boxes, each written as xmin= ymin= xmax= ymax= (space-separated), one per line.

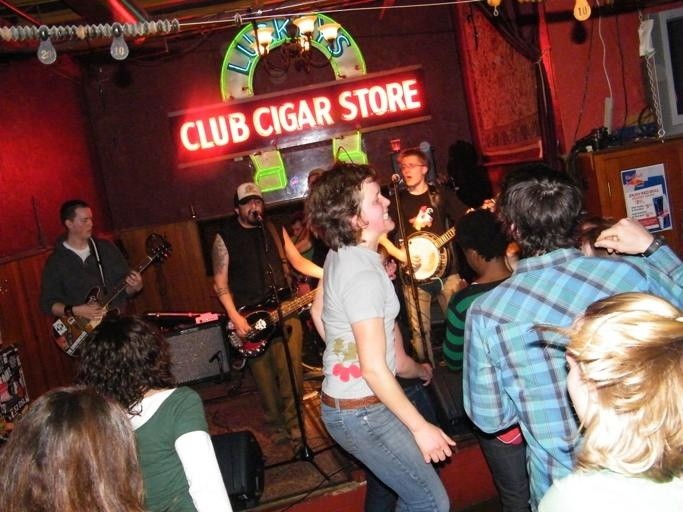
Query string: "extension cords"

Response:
xmin=604 ymin=97 xmax=614 ymax=137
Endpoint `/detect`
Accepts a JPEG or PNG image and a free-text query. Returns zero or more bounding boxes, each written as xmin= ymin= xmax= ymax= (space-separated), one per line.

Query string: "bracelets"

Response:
xmin=64 ymin=305 xmax=74 ymax=319
xmin=643 ymin=233 xmax=668 ymax=257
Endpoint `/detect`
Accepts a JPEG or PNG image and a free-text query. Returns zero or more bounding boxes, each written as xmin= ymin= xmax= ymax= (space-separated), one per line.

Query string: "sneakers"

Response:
xmin=291 ymin=425 xmax=302 ymax=441
xmin=270 ymin=430 xmax=291 ymax=446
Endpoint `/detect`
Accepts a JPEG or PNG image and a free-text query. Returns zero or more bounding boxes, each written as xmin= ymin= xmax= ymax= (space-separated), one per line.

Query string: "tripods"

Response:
xmin=248 ymin=223 xmax=345 ymax=490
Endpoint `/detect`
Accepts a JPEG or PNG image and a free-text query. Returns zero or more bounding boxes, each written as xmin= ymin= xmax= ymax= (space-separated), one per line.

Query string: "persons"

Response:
xmin=304 ymin=164 xmax=456 ymax=511
xmin=462 ymin=161 xmax=683 ymax=512
xmin=580 ymin=216 xmax=619 ymax=231
xmin=40 ymin=199 xmax=145 ymax=362
xmin=210 ymin=181 xmax=324 ymax=458
xmin=0 ymin=381 xmax=147 ymax=512
xmin=380 ymin=148 xmax=497 ymax=368
xmin=534 ymin=292 xmax=683 ymax=511
xmin=578 ymin=224 xmax=628 ymax=258
xmin=376 ymin=244 xmax=446 ymax=437
xmin=441 ymin=207 xmax=533 ymax=512
xmin=73 ymin=314 xmax=237 ymax=512
xmin=286 ymin=209 xmax=314 ymax=284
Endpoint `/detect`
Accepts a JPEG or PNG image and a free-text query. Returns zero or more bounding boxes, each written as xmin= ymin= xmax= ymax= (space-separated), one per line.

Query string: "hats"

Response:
xmin=234 ymin=182 xmax=264 ymax=204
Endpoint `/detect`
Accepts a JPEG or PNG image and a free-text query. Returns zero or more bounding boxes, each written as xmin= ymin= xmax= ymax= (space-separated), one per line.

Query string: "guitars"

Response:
xmin=50 ymin=233 xmax=173 ymax=359
xmin=226 ymin=288 xmax=319 ymax=359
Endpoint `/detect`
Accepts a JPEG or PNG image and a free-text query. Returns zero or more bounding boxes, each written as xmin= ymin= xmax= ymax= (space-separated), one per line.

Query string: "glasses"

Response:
xmin=398 ymin=162 xmax=425 ymax=170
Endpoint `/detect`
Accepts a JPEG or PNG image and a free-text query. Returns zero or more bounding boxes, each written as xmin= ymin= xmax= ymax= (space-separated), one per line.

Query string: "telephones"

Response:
xmin=576 ymin=126 xmax=609 ymax=153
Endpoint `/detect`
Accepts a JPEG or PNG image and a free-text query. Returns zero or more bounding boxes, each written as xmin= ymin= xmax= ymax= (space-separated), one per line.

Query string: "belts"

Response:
xmin=322 ymin=393 xmax=381 ymax=409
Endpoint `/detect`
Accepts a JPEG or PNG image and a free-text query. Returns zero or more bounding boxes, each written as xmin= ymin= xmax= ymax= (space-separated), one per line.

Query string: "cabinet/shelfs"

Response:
xmin=572 ymin=135 xmax=681 ymax=256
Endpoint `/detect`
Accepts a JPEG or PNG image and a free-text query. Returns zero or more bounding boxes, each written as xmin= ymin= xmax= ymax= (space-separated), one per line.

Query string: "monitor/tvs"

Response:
xmin=650 ymin=6 xmax=683 ymax=135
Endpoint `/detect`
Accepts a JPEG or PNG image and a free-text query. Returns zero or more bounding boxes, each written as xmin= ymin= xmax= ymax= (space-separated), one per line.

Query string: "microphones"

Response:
xmin=251 ymin=208 xmax=263 ymax=224
xmin=391 ymin=173 xmax=401 ymax=184
xmin=209 ymin=350 xmax=220 ymax=363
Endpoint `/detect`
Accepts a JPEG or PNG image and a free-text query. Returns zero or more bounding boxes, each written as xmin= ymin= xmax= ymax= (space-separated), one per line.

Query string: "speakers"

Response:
xmin=429 ymin=366 xmax=474 ymax=432
xmin=210 ymin=430 xmax=264 ymax=510
xmin=150 ymin=324 xmax=234 ymax=386
